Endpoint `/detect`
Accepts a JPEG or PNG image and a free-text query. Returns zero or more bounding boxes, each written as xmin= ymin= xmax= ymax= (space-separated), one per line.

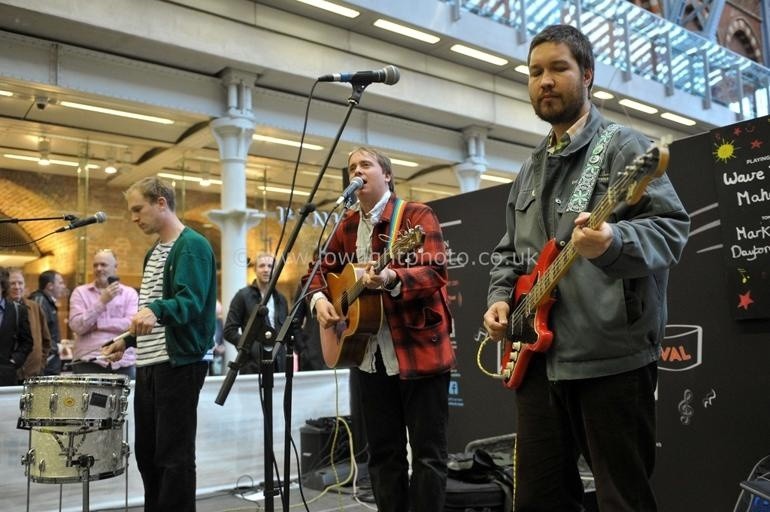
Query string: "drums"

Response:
xmin=16 ymin=374 xmax=131 ymax=427
xmin=23 ymin=427 xmax=130 ymax=483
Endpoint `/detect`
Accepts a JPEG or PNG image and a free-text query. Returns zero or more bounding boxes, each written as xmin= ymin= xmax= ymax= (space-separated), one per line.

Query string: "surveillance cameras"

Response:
xmin=35 ymin=97 xmax=48 ymax=111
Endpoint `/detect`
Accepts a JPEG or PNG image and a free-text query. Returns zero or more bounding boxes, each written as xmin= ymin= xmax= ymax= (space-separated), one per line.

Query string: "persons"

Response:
xmin=27 ymin=269 xmax=66 ymax=376
xmin=6 ymin=268 xmax=53 ymax=385
xmin=0 ymin=265 xmax=34 ymax=387
xmin=297 ymin=146 xmax=457 ymax=512
xmin=221 ymin=254 xmax=290 ymax=375
xmin=484 ymin=24 xmax=691 ymax=510
xmin=101 ymin=176 xmax=218 ymax=512
xmin=67 ymin=247 xmax=139 ymax=380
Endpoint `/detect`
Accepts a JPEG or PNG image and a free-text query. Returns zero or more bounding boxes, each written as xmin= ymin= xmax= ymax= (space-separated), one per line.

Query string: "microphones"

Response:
xmin=56 ymin=211 xmax=107 ymax=232
xmin=318 ymin=64 xmax=401 ymax=85
xmin=333 ymin=176 xmax=364 ymax=208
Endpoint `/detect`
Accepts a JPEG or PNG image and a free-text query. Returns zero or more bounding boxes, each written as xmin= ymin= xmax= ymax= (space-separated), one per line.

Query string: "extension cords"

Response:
xmin=306 ymin=424 xmax=327 ymax=432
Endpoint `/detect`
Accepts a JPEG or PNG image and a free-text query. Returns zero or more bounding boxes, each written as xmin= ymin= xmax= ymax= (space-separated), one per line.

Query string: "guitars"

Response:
xmin=476 ymin=137 xmax=672 ymax=388
xmin=319 ymin=225 xmax=426 ymax=368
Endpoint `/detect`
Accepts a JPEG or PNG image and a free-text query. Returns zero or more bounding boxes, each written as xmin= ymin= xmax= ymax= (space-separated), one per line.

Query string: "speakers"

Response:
xmin=302 ymin=424 xmax=354 ymax=475
